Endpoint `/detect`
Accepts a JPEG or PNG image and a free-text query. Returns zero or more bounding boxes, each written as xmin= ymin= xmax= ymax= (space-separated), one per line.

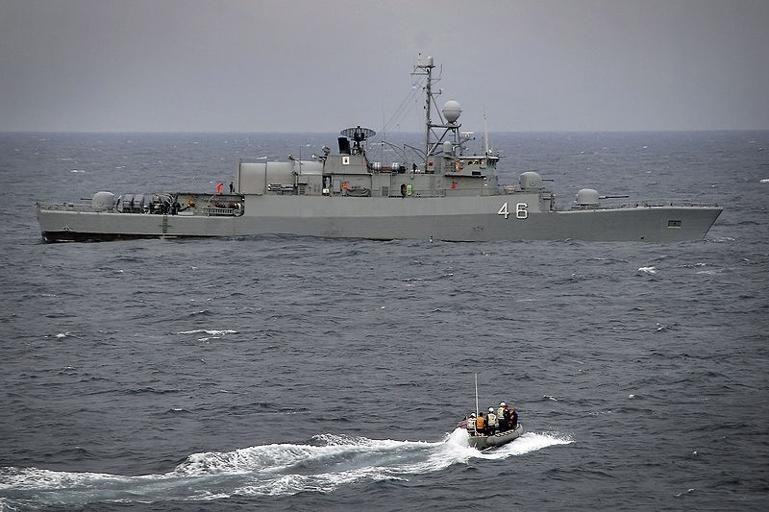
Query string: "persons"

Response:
xmin=229 ymin=181 xmax=233 ymax=192
xmin=216 ymin=181 xmax=223 ymax=193
xmin=464 ymin=400 xmax=519 ymax=436
xmin=413 ymin=162 xmax=417 ymax=174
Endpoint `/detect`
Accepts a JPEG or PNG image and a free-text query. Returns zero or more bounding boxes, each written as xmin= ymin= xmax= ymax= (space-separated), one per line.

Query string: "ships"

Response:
xmin=34 ymin=52 xmax=724 ymax=246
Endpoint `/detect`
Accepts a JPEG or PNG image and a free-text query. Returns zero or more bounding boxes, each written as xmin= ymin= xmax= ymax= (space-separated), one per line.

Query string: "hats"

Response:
xmin=499 ymin=402 xmax=507 ymax=407
xmin=470 ymin=412 xmax=476 ymax=418
xmin=487 ymin=406 xmax=494 ymax=413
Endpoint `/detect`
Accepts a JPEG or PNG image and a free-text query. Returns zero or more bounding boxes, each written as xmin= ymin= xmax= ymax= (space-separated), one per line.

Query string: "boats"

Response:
xmin=465 ymin=373 xmax=523 ymax=449
